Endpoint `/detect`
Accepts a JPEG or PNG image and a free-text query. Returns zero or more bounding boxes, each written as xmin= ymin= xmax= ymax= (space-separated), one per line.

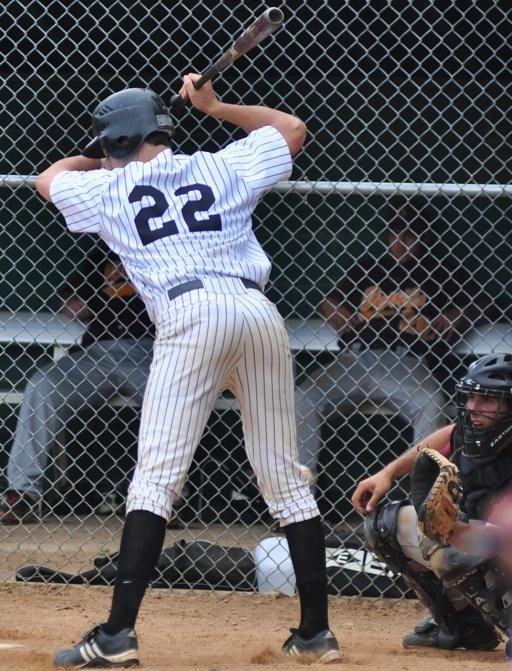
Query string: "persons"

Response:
xmin=0 ymin=237 xmax=189 ymax=529
xmin=352 ymin=352 xmax=512 ymax=658
xmin=270 ymin=196 xmax=465 ymax=532
xmin=35 ymin=72 xmax=342 ymax=668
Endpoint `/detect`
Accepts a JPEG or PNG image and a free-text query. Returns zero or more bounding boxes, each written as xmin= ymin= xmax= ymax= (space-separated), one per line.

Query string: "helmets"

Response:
xmin=81 ymin=88 xmax=176 ymax=159
xmin=454 ymin=353 xmax=511 ymax=458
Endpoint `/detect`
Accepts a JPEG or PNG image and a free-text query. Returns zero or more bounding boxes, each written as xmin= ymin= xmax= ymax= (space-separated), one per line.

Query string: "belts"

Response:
xmin=168 ymin=277 xmax=262 ymax=301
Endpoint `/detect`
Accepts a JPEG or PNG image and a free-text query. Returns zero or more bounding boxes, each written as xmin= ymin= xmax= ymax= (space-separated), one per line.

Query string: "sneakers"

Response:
xmin=282 ymin=627 xmax=340 ymax=664
xmin=401 ymin=618 xmax=502 ymax=651
xmin=1 ymin=489 xmax=40 ymax=522
xmin=54 ymin=622 xmax=140 ymax=669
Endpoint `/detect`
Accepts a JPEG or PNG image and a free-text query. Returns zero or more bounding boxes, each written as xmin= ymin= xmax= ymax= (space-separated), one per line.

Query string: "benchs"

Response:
xmin=0 ymin=309 xmax=511 ymax=504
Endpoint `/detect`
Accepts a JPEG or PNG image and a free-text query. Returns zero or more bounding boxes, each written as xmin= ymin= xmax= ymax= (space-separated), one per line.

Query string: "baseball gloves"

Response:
xmin=410 ymin=448 xmax=462 ymax=545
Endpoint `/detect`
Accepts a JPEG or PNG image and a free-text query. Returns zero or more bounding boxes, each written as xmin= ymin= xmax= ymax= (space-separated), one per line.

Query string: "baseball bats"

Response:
xmin=169 ymin=7 xmax=285 ymax=109
